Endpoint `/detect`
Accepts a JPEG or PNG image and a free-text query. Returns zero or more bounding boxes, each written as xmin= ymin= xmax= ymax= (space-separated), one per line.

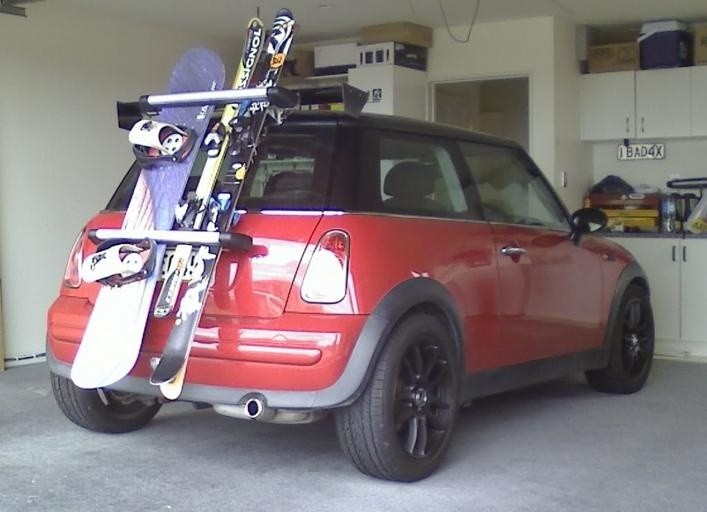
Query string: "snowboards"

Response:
xmin=71 ymin=47 xmax=226 ymax=390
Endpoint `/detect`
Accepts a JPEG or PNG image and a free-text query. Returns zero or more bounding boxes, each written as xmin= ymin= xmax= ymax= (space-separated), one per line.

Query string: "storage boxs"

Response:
xmin=590 ymin=20 xmax=706 ymax=71
xmin=281 ymin=22 xmax=432 ymax=76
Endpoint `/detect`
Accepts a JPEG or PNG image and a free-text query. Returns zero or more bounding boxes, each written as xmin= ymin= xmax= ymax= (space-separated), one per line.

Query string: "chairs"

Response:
xmin=184 ymin=161 xmax=448 ymax=215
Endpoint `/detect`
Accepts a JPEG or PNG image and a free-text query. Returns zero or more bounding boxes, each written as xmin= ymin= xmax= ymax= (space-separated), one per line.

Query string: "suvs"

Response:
xmin=46 ymin=85 xmax=654 ymax=483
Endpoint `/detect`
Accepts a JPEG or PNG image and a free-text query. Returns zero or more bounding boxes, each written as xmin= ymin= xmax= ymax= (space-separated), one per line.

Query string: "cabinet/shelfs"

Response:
xmin=606 ymin=237 xmax=707 ymax=367
xmin=582 ymin=67 xmax=692 ymax=142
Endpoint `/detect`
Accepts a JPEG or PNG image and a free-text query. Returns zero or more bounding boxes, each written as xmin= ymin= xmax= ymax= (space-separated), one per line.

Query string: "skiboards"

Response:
xmin=150 ymin=7 xmax=299 ymax=400
xmin=154 ymin=17 xmax=272 ymax=320
